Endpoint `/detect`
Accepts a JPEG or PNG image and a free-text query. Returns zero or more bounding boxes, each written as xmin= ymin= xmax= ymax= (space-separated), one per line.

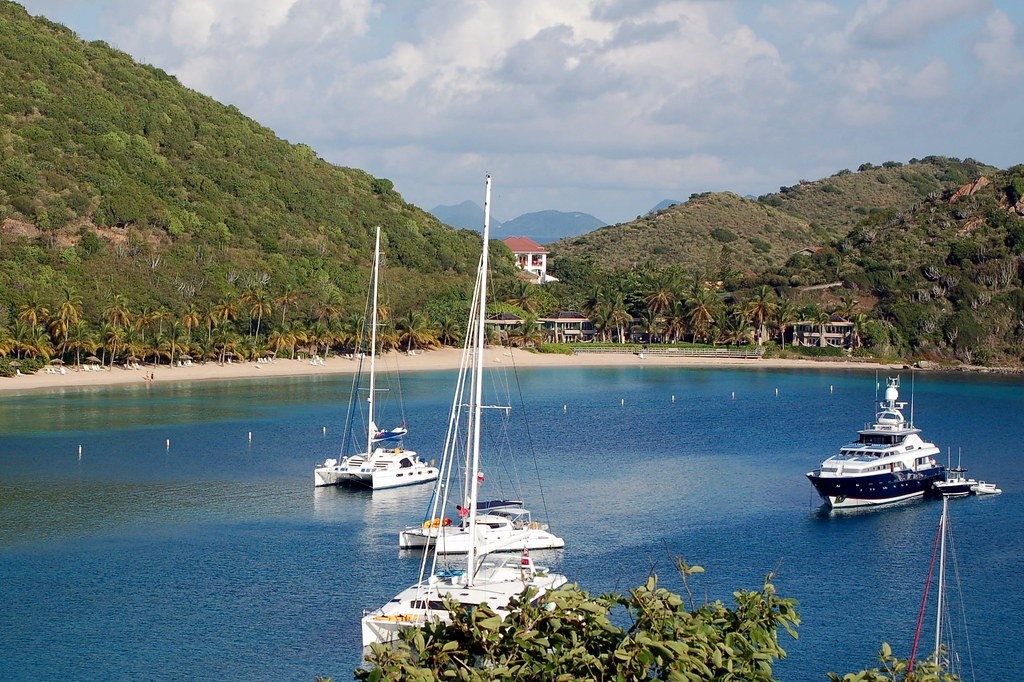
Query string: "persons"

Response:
xmin=146 ymin=371 xmax=149 ymax=380
xmin=430 ymin=456 xmax=435 ymax=467
xmin=930 ymin=458 xmax=936 ymax=469
xmin=150 ymin=372 xmax=154 ymax=384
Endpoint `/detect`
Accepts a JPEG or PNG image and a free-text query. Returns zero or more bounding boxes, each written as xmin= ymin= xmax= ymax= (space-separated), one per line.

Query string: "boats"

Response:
xmin=929 ymin=445 xmax=978 ymax=498
xmin=804 ymin=367 xmax=949 ymax=511
xmin=971 ymin=481 xmax=1004 ymax=495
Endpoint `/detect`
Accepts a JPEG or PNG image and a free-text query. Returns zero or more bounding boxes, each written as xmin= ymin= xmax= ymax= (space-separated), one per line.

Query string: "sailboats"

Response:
xmin=362 ymin=172 xmax=575 ymax=650
xmin=312 ymin=220 xmax=440 ymax=491
xmin=396 ymin=248 xmax=566 ymax=553
